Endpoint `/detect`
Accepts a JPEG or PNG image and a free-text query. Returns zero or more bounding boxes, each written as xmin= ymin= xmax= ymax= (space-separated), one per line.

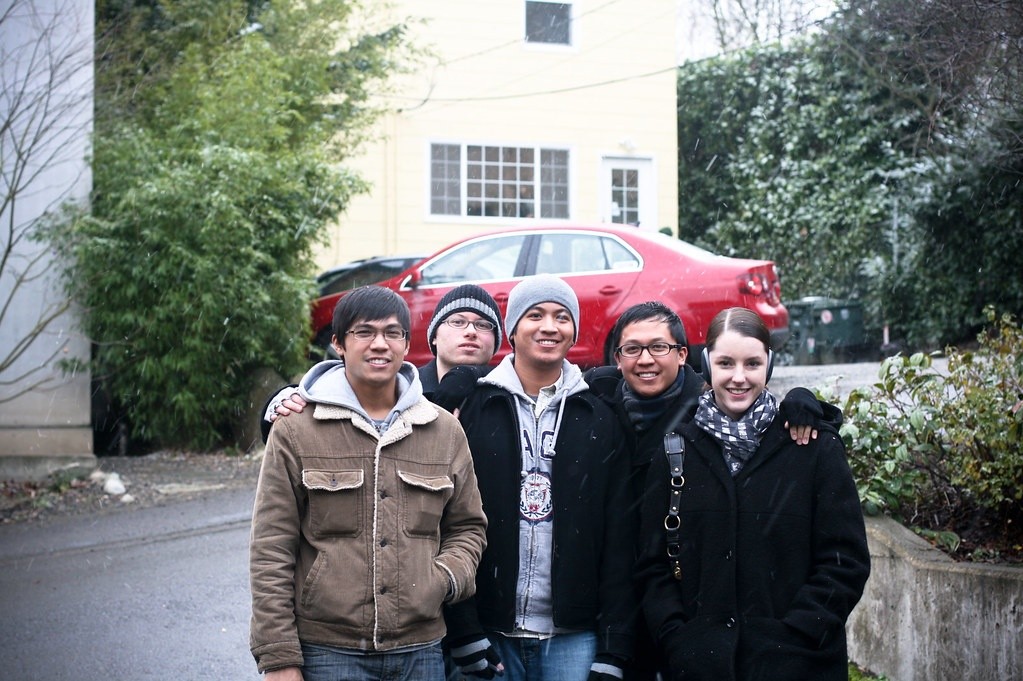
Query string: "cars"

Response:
xmin=311 ymin=221 xmax=790 ymax=375
xmin=314 ymin=254 xmax=496 ymax=298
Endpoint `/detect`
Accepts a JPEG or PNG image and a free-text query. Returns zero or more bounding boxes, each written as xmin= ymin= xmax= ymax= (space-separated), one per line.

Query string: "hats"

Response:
xmin=427 ymin=284 xmax=503 ymax=358
xmin=505 ymin=274 xmax=580 ymax=348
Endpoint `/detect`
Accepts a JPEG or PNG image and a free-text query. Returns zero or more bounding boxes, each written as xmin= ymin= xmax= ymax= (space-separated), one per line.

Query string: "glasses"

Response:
xmin=615 ymin=341 xmax=683 ymax=358
xmin=441 ymin=318 xmax=497 ymax=332
xmin=344 ymin=327 xmax=409 ymax=341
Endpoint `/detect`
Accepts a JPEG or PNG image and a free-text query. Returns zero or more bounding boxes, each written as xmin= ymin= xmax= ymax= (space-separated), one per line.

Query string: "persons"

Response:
xmin=250 ymin=283 xmax=488 ymax=681
xmin=585 ymin=303 xmax=825 ymax=681
xmin=260 ymin=285 xmax=523 ymax=681
xmin=443 ymin=272 xmax=640 ymax=681
xmin=641 ymin=306 xmax=872 ymax=681
